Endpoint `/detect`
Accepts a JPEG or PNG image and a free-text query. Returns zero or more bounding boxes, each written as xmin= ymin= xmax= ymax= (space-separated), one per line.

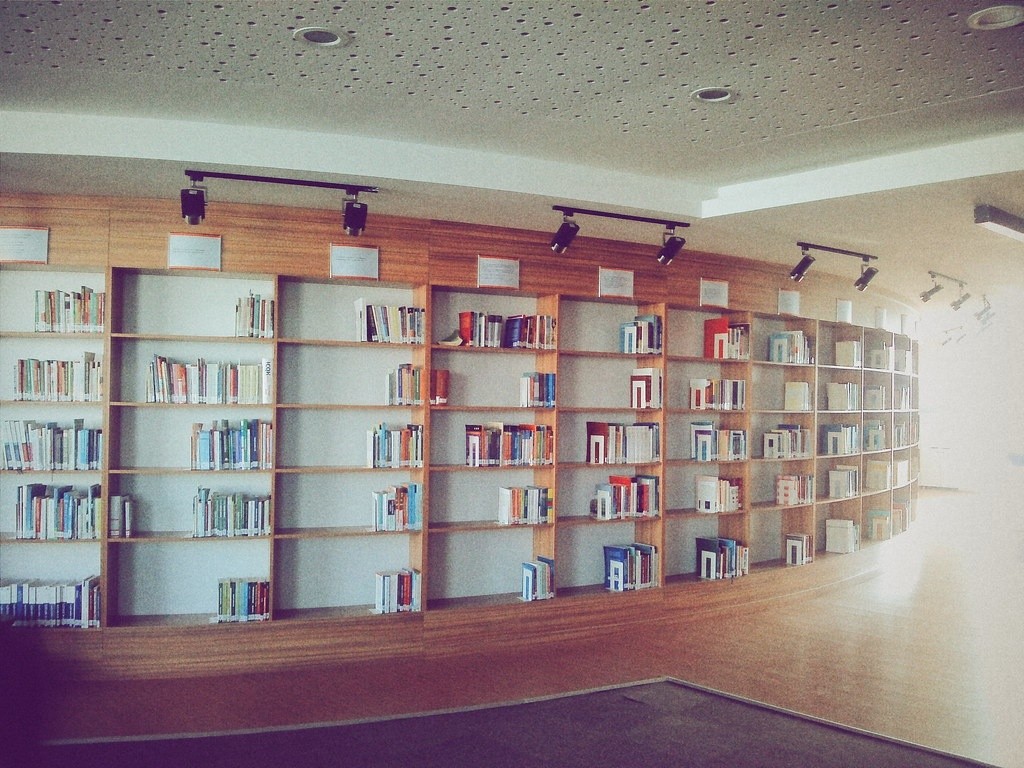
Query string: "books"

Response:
xmin=603 ymin=542 xmax=661 ymax=591
xmin=619 ymin=313 xmax=662 ymax=356
xmin=768 ymin=328 xmax=816 ymax=364
xmin=784 ymin=382 xmax=813 ymax=411
xmin=354 ymin=296 xmax=426 ymax=345
xmin=589 ymin=473 xmax=659 ymax=521
xmin=145 ymin=352 xmax=275 ymax=405
xmin=690 ymin=422 xmax=749 ymax=461
xmin=826 ymin=330 xmax=912 ymax=555
xmin=429 ymin=367 xmax=449 ymax=406
xmin=192 ymin=486 xmax=271 ymax=538
xmin=776 ymin=472 xmax=814 ymax=506
xmin=520 ymin=555 xmax=555 ymax=602
xmin=464 ymin=421 xmax=553 ymax=467
xmin=367 ymin=419 xmax=424 ymax=469
xmin=584 ymin=420 xmax=661 ymax=464
xmin=435 ymin=311 xmax=556 ymax=349
xmin=688 ymin=377 xmax=746 ymax=410
xmin=108 ymin=493 xmax=136 ymax=539
xmin=34 ymin=286 xmax=104 ymax=334
xmin=786 ymin=532 xmax=814 ymax=565
xmin=234 ymin=288 xmax=275 ymax=339
xmin=386 ymin=362 xmax=424 ymax=406
xmin=375 ymin=567 xmax=423 ymax=614
xmin=14 ymin=352 xmax=104 ymax=402
xmin=703 ymin=317 xmax=750 ymax=361
xmin=16 ymin=483 xmax=101 ymax=539
xmin=190 ymin=418 xmax=273 ymax=471
xmin=371 ymin=481 xmax=423 ymax=532
xmin=0 ymin=419 xmax=102 ymax=470
xmin=497 ymin=486 xmax=554 ymax=525
xmin=0 ymin=575 xmax=102 ymax=629
xmin=218 ymin=576 xmax=270 ymax=622
xmin=695 ymin=474 xmax=743 ymax=514
xmin=630 ymin=366 xmax=663 ymax=410
xmin=763 ymin=423 xmax=812 ymax=459
xmin=520 ymin=372 xmax=555 ymax=408
xmin=696 ymin=537 xmax=748 ymax=580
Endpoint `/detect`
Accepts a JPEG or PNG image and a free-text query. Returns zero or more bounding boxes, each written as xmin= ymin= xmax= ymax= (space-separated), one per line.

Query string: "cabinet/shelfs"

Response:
xmin=0 ymin=187 xmax=923 ymax=684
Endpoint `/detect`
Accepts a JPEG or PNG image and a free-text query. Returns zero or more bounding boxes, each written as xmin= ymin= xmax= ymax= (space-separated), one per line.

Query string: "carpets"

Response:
xmin=37 ymin=675 xmax=995 ymax=768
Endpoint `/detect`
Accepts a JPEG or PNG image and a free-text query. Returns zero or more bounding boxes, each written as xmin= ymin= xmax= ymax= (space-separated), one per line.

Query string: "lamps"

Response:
xmin=950 ymin=292 xmax=971 ymax=311
xmin=853 ymin=262 xmax=879 ymax=291
xmin=180 ymin=180 xmax=209 ymax=226
xmin=550 ymin=214 xmax=581 ymax=255
xmin=656 ymin=229 xmax=686 ymax=267
xmin=341 ymin=193 xmax=368 ymax=237
xmin=974 ymin=306 xmax=996 ymax=326
xmin=975 ymin=204 xmax=1024 ymax=243
xmin=789 ymin=250 xmax=816 ymax=282
xmin=920 ymin=284 xmax=945 ymax=303
